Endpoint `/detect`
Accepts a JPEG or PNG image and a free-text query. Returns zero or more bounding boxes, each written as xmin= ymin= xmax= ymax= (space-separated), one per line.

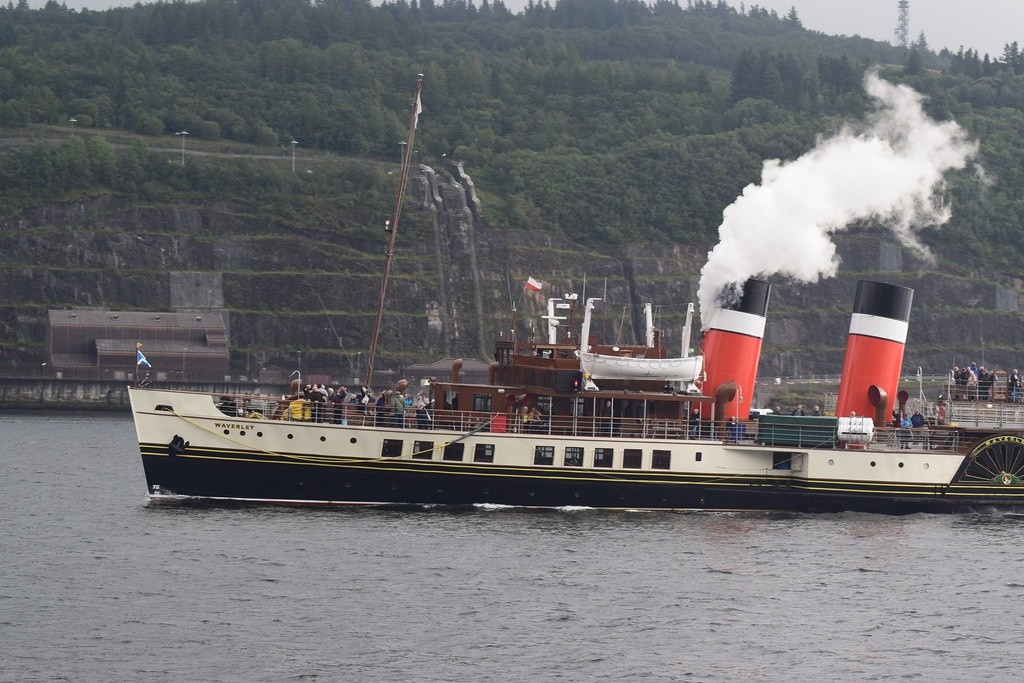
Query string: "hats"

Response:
xmin=954 ymin=367 xmax=958 ymax=371
xmin=962 ymin=368 xmax=966 ymax=372
xmin=915 ymin=408 xmax=920 ymax=411
xmin=395 ymin=391 xmax=400 ymax=398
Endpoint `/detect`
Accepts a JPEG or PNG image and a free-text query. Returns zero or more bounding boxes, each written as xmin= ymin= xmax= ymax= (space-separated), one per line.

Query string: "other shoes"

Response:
xmin=905 ymin=447 xmax=911 ymax=449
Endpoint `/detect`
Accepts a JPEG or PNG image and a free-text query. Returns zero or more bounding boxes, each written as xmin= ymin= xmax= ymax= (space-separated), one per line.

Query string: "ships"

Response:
xmin=124 ymin=69 xmax=1023 ymax=516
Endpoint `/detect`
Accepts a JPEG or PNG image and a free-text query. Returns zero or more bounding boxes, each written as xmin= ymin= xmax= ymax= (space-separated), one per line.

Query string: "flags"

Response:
xmin=525 ymin=275 xmax=542 ymax=292
xmin=413 ymin=92 xmax=422 ymax=130
xmin=137 ymin=349 xmax=151 ymax=368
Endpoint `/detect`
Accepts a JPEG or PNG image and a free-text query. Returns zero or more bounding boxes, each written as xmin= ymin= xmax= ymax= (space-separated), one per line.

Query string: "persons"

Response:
xmin=303 ymin=383 xmax=459 ymax=429
xmin=726 ymin=416 xmax=736 ymax=440
xmin=791 ymin=405 xmax=805 ymax=416
xmin=910 ymin=410 xmax=924 ymax=445
xmin=687 ymin=409 xmax=699 ymax=440
xmin=893 ymin=414 xmax=902 ymax=437
xmin=948 ymin=360 xmax=1024 ymax=400
xmin=521 ymin=405 xmax=543 ymax=434
xmin=811 ymin=406 xmax=822 ymax=416
xmin=899 ymin=413 xmax=912 ymax=449
xmin=772 ymin=406 xmax=782 ymax=415
